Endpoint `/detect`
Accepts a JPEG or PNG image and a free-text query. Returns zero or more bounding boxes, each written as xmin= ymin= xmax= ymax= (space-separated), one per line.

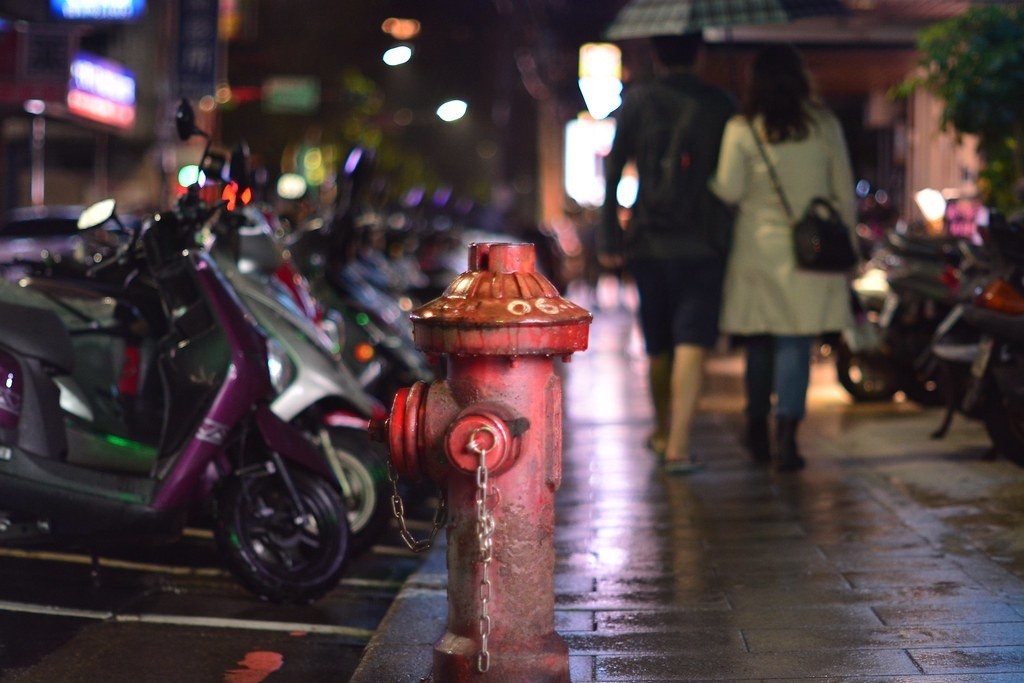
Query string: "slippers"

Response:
xmin=664 ymin=450 xmax=706 ymax=474
xmin=647 ymin=438 xmax=667 ymax=466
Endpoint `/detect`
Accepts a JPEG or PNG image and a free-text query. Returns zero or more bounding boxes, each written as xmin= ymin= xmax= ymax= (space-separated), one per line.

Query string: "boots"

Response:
xmin=741 ymin=407 xmax=770 ymax=462
xmin=775 ymin=413 xmax=805 ymax=471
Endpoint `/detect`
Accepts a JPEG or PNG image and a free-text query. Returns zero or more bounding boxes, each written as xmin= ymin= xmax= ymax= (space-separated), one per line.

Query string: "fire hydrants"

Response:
xmin=382 ymin=240 xmax=595 ymax=682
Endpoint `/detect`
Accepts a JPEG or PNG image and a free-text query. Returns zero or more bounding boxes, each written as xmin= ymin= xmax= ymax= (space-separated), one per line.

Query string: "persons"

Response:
xmin=599 ymin=37 xmax=738 ymax=468
xmin=709 ymin=40 xmax=860 ymax=473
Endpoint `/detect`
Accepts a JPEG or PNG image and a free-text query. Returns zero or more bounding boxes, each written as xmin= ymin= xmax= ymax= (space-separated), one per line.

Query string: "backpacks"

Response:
xmin=631 ymin=84 xmax=697 ymax=230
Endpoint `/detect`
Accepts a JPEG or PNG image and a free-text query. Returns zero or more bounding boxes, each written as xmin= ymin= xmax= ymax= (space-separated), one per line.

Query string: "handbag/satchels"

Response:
xmin=791 ymin=196 xmax=858 ymax=273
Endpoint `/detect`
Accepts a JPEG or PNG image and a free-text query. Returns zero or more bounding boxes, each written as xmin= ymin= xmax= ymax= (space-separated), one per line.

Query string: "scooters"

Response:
xmin=1 ymin=93 xmax=567 ymax=606
xmin=834 ymin=223 xmax=1023 ymax=471
